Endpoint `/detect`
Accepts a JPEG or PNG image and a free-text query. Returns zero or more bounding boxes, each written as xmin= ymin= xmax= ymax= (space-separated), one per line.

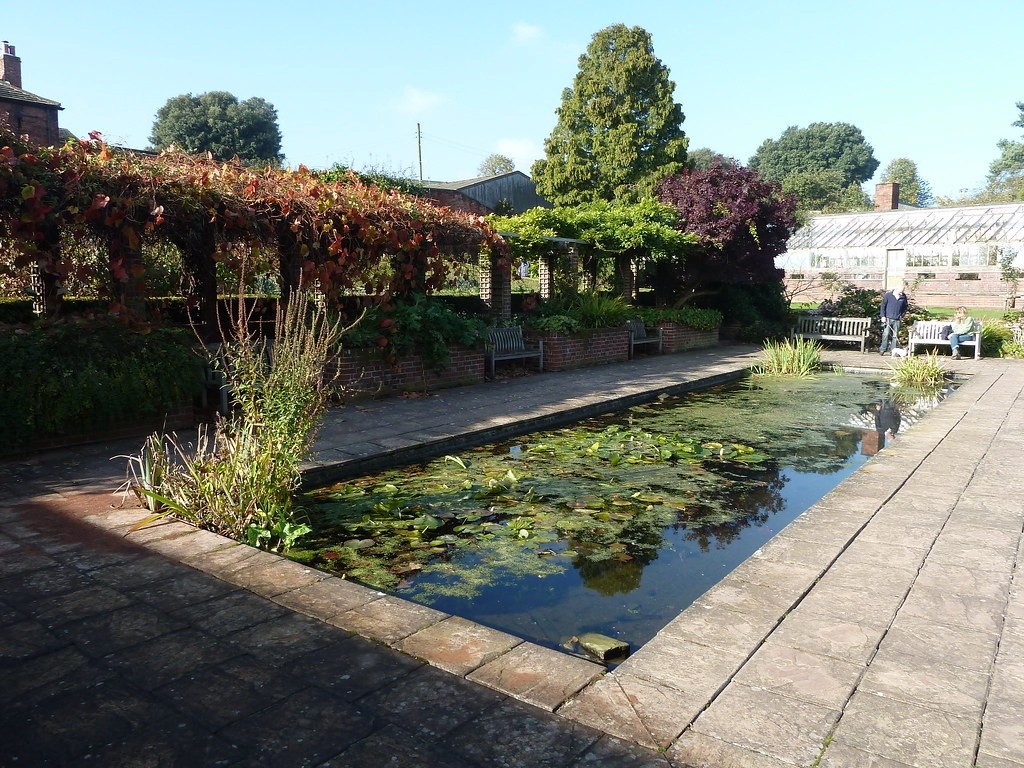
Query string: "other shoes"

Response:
xmin=879 ymin=350 xmax=883 ymax=354
xmin=952 ymin=351 xmax=961 ymax=360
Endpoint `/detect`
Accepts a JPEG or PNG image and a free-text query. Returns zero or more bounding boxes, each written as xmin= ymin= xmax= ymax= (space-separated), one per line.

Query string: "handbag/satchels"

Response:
xmin=941 ymin=325 xmax=953 ymax=340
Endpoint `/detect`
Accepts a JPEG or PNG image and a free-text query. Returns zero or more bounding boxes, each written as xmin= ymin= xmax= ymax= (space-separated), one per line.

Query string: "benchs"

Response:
xmin=474 ymin=325 xmax=544 ymax=377
xmin=622 ymin=319 xmax=663 ymax=360
xmin=193 ymin=339 xmax=284 ymax=414
xmin=790 ymin=315 xmax=872 ymax=354
xmin=907 ymin=320 xmax=982 ymax=361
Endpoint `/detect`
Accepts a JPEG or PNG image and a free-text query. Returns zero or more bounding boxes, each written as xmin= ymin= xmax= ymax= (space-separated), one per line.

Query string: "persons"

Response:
xmin=947 ymin=306 xmax=975 ymax=361
xmin=879 ymin=279 xmax=908 ymax=356
